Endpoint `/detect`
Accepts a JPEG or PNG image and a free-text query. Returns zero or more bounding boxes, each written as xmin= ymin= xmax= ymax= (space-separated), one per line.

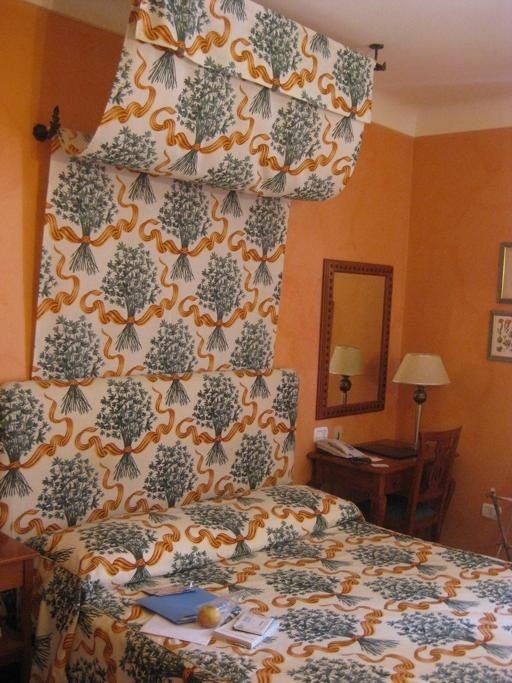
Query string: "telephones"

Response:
xmin=315 ymin=438 xmax=364 ymax=459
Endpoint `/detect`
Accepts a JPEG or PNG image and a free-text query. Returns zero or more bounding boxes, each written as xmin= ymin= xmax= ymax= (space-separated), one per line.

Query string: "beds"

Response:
xmin=0 ymin=368 xmax=512 ymax=683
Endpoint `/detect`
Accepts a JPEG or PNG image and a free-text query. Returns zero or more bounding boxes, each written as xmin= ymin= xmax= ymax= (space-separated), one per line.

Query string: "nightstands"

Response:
xmin=0 ymin=532 xmax=39 ymax=683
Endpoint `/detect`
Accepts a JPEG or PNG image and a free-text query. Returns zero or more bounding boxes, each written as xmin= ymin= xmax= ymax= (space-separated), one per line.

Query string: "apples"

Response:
xmin=198 ymin=605 xmax=220 ymax=628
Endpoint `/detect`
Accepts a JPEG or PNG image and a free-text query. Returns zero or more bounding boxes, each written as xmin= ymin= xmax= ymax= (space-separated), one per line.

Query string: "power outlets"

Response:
xmin=314 ymin=427 xmax=329 ymax=443
xmin=481 ymin=503 xmax=503 ymax=520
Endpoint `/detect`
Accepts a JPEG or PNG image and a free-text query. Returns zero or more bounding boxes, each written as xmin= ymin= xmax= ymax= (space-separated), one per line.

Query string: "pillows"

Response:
xmin=25 ymin=485 xmax=390 ymax=589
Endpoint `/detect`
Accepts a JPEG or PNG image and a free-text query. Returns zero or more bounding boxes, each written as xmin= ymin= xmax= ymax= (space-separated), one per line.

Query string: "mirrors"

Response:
xmin=314 ymin=258 xmax=393 ymax=420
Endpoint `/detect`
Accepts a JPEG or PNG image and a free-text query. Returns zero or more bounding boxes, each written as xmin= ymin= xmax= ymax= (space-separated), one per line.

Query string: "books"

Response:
xmin=235 ymin=613 xmax=273 ymax=636
xmin=141 ymin=587 xmax=219 ymax=625
xmin=216 ymin=612 xmax=279 ymax=649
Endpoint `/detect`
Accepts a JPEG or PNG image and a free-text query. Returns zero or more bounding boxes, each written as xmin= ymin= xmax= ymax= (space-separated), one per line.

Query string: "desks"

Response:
xmin=306 ymin=438 xmax=460 ymax=542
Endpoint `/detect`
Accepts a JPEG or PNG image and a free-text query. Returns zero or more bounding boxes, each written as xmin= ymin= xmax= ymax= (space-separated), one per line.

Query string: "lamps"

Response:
xmin=329 ymin=344 xmax=363 ymax=403
xmin=392 ymin=353 xmax=450 ymax=450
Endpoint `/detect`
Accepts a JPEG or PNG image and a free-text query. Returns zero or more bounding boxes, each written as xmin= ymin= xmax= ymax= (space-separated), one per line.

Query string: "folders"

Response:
xmin=133 ymin=588 xmax=242 ymax=625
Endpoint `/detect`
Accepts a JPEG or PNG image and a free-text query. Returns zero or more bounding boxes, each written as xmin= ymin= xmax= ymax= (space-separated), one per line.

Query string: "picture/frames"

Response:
xmin=486 ymin=309 xmax=512 ymax=363
xmin=498 ymin=242 xmax=511 ymax=304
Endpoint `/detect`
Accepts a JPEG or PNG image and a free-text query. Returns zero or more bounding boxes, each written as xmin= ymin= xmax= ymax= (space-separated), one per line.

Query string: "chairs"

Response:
xmin=386 ymin=425 xmax=463 ymax=543
